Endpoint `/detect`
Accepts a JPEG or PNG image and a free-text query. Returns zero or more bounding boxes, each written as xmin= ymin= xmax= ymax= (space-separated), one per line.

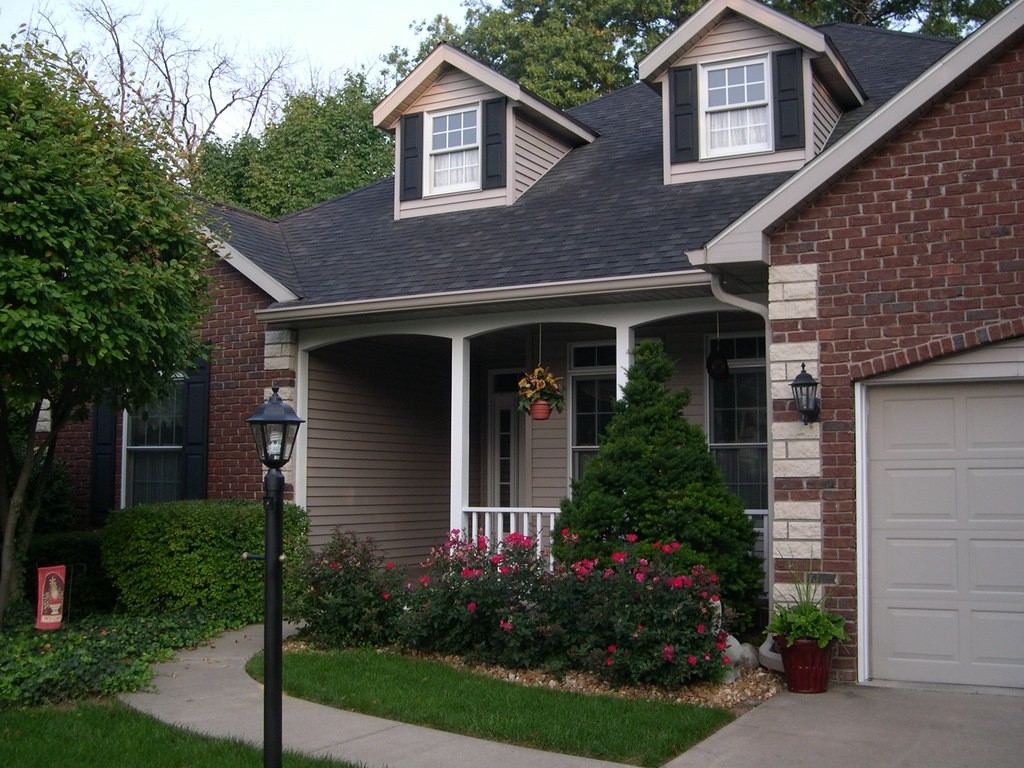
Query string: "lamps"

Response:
xmin=787 ymin=362 xmax=821 ymax=427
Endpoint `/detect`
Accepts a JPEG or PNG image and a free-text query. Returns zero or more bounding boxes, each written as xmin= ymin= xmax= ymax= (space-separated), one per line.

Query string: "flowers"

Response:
xmin=518 ymin=365 xmax=566 ymax=416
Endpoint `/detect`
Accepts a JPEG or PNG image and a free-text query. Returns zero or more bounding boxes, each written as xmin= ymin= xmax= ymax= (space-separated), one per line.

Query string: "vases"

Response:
xmin=529 ymin=399 xmax=551 ymax=420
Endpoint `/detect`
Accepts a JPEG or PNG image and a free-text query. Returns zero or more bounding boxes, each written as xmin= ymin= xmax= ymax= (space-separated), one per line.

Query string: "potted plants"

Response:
xmin=761 ymin=544 xmax=849 ymax=693
